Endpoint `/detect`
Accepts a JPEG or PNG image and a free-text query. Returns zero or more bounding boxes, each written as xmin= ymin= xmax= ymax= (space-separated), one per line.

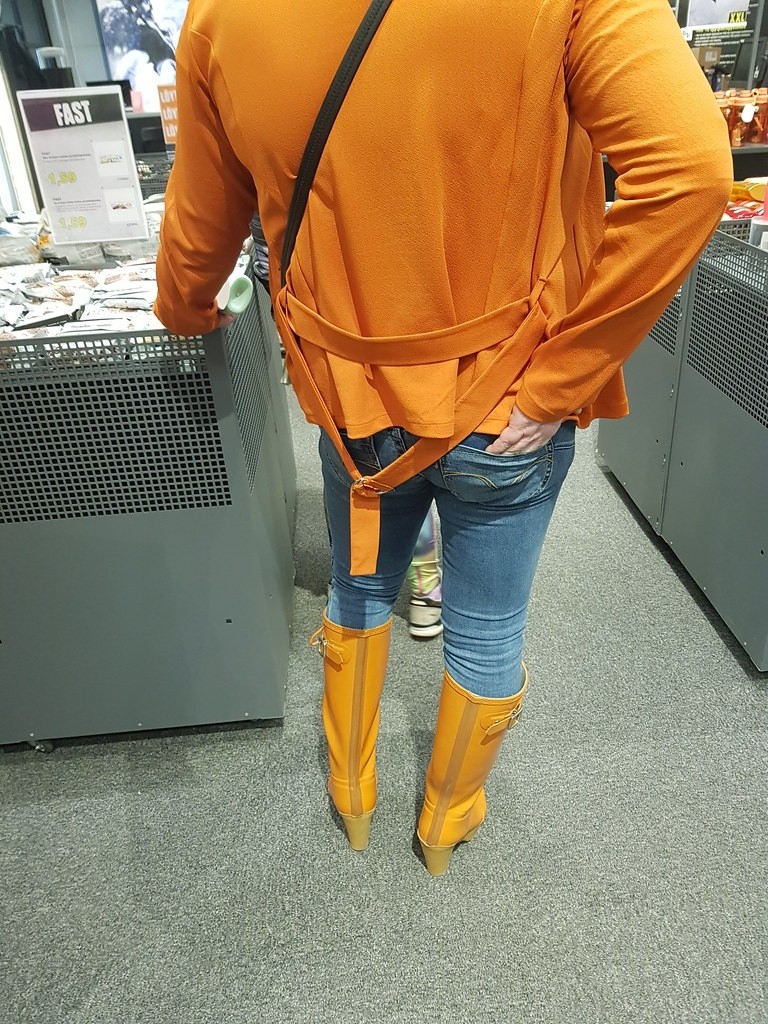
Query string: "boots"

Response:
xmin=417 ymin=661 xmax=529 ymax=877
xmin=310 ymin=605 xmax=393 ymax=850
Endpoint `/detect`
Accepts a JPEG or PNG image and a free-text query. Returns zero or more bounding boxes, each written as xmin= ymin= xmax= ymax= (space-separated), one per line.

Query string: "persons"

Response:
xmin=152 ymin=1 xmax=734 ymax=876
xmin=406 ymin=507 xmax=445 ymax=638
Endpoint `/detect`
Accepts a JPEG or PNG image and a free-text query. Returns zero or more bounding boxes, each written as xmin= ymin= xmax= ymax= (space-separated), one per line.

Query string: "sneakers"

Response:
xmin=409 ymin=597 xmax=444 ymax=636
